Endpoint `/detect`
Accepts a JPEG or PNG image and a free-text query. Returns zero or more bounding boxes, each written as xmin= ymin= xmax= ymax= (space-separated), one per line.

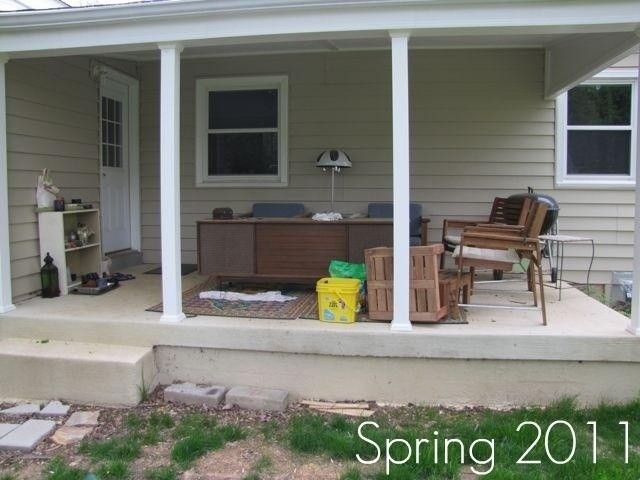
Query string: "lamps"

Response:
xmin=314 ymin=145 xmax=352 ymax=216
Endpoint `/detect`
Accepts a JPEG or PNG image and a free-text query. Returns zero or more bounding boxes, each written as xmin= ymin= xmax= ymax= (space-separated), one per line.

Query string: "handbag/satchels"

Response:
xmin=37 ymin=168 xmax=56 ymax=208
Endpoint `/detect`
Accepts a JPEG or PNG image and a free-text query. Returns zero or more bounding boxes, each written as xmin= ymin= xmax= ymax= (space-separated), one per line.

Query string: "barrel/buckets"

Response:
xmin=316 ymin=277 xmax=363 ymax=323
xmin=330 ymin=259 xmax=367 ymax=315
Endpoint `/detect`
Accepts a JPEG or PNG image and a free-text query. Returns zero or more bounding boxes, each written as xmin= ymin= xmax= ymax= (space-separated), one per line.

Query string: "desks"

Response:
xmin=535 ymin=233 xmax=595 ymax=302
xmin=193 ymin=217 xmax=431 ymax=286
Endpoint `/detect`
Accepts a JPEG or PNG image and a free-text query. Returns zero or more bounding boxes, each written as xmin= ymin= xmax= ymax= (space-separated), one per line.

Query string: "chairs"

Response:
xmin=254 ymin=200 xmax=422 ymax=246
xmin=440 ymin=196 xmax=549 ymax=326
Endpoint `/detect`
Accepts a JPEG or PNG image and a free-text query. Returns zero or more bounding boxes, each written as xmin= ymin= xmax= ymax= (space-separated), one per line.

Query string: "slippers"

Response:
xmin=113 ymin=272 xmax=135 ymax=280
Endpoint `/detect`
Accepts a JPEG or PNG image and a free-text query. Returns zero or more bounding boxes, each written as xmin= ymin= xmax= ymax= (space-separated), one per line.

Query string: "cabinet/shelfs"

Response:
xmin=36 ymin=209 xmax=104 ymax=297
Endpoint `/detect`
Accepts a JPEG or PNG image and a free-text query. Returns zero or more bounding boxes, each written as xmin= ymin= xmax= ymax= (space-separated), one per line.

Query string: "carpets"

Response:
xmin=143 ymin=263 xmax=198 ymax=275
xmin=144 ymin=278 xmax=386 ymax=323
